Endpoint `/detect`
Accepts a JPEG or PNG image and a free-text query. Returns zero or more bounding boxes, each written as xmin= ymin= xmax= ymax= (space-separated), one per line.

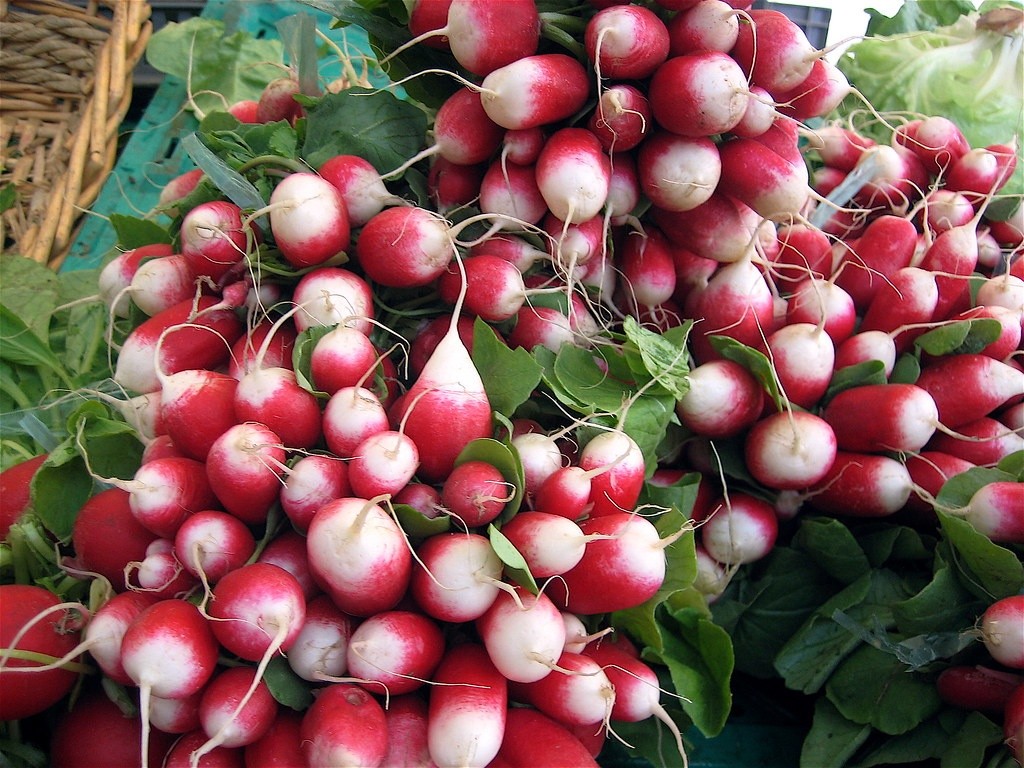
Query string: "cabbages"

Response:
xmin=829 ymin=0 xmax=1024 ymax=225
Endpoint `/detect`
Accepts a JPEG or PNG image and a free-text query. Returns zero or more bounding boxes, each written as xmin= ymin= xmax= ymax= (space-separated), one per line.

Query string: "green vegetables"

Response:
xmin=0 ymin=2 xmax=1024 ymax=768
